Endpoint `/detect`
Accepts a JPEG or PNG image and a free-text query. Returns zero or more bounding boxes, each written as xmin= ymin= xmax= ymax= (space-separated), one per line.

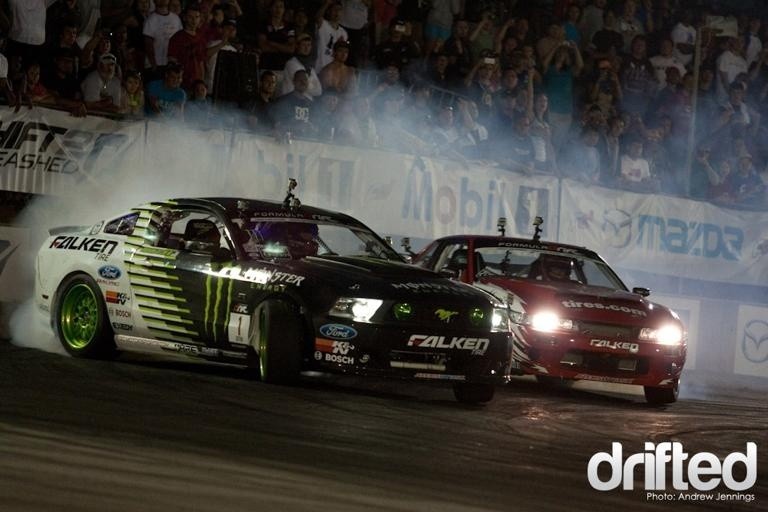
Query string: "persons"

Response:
xmin=0 ymin=0 xmax=768 ymax=210
xmin=535 ymin=258 xmax=574 ymax=282
xmin=287 ymin=222 xmax=319 ymax=259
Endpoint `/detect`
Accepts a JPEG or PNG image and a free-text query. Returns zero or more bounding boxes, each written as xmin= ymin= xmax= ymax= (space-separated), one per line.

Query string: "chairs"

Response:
xmin=447 ymin=248 xmax=485 ymax=272
xmin=184 ymin=219 xmax=221 ymax=244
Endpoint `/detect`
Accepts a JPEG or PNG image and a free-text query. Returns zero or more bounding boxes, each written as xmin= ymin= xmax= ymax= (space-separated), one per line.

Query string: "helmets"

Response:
xmin=540 ymin=254 xmax=571 ymax=281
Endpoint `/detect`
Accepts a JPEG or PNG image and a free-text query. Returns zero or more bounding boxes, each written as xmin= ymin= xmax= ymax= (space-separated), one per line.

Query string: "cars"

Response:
xmin=409 ymin=235 xmax=686 ymax=403
xmin=36 ymin=195 xmax=513 ymax=404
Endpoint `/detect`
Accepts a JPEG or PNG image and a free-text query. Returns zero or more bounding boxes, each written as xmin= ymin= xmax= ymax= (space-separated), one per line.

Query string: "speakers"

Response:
xmin=211 ymin=49 xmax=260 ymax=116
